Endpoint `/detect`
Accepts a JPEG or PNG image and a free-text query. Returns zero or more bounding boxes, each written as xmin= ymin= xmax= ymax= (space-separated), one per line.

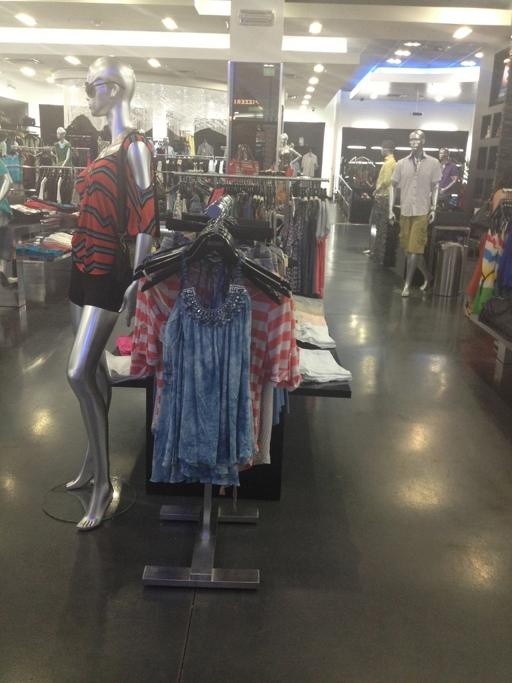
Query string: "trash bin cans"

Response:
xmin=431 ymin=242 xmax=465 ymax=298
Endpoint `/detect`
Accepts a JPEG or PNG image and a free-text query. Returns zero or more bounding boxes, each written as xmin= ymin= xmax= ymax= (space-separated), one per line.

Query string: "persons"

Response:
xmin=3 ymin=141 xmax=21 ymax=182
xmin=388 ymin=129 xmax=444 ymax=297
xmin=162 ymin=137 xmax=174 ymax=154
xmin=368 ymin=140 xmax=396 ymax=266
xmin=65 ymin=55 xmax=158 ymax=531
xmin=0 ymin=158 xmax=14 ymax=288
xmin=50 ymin=127 xmax=72 ymax=166
xmin=437 ymin=148 xmax=459 ymax=207
xmin=278 ymin=133 xmax=302 ymax=176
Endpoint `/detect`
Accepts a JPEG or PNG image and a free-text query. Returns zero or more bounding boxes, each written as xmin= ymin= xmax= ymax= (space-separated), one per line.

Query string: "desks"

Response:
xmin=109 ymin=340 xmax=351 ymax=501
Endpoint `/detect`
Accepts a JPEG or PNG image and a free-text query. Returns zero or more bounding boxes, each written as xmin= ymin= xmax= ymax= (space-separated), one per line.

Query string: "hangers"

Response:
xmin=489 ymin=188 xmax=512 ymax=229
xmin=39 ymin=155 xmax=333 ymax=304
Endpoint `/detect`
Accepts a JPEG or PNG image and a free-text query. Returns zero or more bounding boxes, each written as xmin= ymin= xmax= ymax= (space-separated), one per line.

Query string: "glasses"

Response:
xmin=85 ymin=80 xmax=125 ymax=98
